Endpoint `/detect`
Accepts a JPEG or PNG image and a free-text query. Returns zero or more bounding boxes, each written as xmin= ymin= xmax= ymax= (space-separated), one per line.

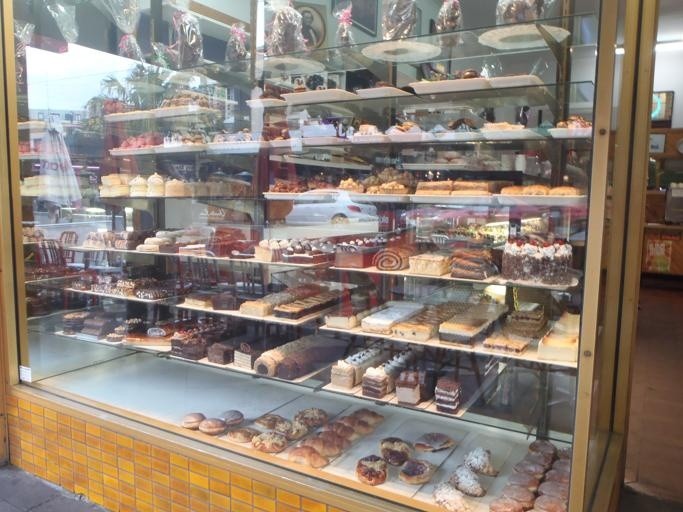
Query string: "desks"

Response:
xmin=642 ymin=222 xmax=683 ymax=274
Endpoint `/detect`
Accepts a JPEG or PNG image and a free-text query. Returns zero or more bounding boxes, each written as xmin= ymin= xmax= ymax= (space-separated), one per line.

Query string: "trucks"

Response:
xmin=17 ymin=204 xmax=127 ymax=273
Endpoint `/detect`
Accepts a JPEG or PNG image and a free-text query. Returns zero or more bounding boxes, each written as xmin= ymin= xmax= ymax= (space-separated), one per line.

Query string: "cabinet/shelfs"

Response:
xmin=0 ymin=1 xmax=658 ymax=511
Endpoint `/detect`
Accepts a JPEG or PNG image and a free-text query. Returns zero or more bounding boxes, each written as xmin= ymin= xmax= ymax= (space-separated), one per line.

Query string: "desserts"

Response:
xmin=64 ymin=118 xmax=593 ymax=512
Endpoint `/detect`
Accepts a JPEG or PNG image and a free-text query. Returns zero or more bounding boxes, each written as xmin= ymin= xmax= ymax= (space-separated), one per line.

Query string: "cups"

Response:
xmin=501 ymin=152 xmax=552 ymax=179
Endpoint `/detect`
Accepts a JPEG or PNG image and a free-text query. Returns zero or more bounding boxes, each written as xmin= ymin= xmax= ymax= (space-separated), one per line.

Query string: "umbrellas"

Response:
xmin=34 ymin=109 xmax=82 ymax=223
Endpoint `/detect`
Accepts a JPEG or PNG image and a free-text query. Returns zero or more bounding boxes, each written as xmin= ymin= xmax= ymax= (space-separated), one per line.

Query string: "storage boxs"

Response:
xmin=645 ymin=188 xmax=667 ymax=224
xmin=648 ymin=127 xmax=683 ymax=154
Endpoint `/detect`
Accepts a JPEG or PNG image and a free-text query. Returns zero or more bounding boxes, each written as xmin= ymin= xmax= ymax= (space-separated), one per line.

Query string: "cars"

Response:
xmin=392 ymin=196 xmax=584 ymax=245
xmin=276 ymin=184 xmax=378 ymax=229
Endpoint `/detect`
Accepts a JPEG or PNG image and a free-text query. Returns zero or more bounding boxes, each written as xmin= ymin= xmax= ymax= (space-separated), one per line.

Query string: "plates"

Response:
xmin=478 ymin=24 xmax=571 ymax=50
xmin=262 ymin=59 xmax=324 ymax=75
xmin=360 ymin=41 xmax=442 ymax=63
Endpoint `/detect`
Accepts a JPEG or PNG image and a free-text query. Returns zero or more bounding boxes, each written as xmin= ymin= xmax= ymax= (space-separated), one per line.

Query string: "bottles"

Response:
xmin=73 ymin=114 xmax=80 ymax=125
